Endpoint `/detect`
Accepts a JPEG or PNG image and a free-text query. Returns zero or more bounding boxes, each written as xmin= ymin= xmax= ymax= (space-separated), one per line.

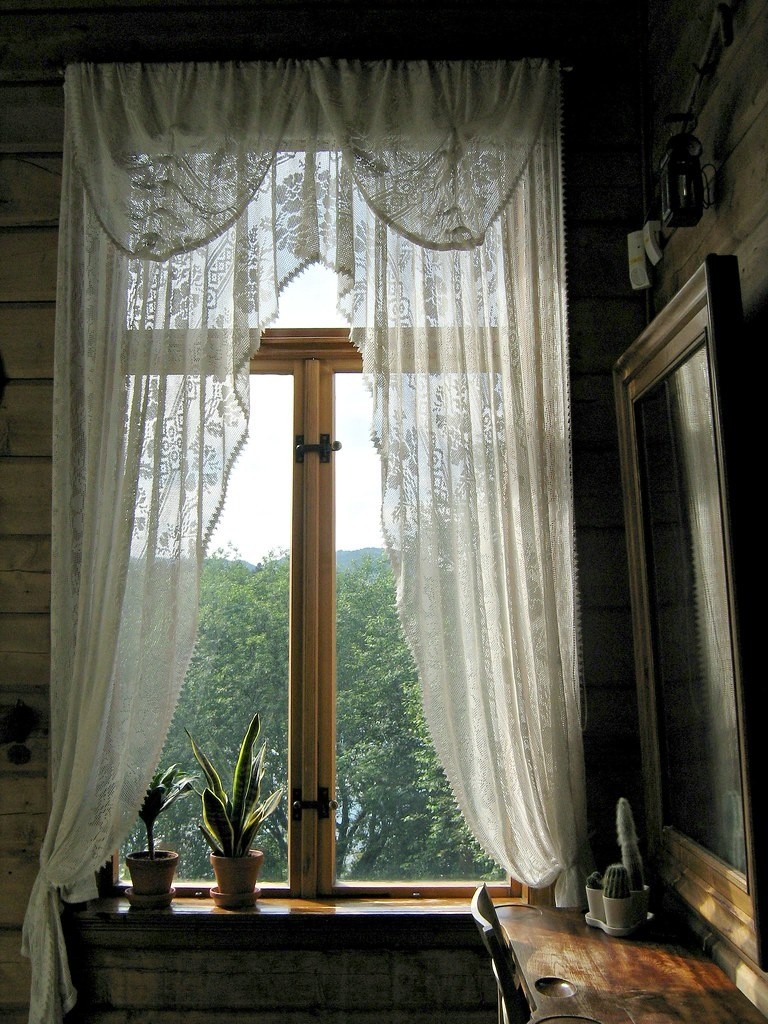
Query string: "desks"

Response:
xmin=71 ymin=893 xmax=768 ymax=1024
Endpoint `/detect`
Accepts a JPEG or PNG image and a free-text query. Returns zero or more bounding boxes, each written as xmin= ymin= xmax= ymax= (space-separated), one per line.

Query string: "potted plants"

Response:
xmin=123 ymin=763 xmax=201 ymax=908
xmin=184 ymin=712 xmax=286 ymax=908
xmin=583 ymin=795 xmax=655 ymax=938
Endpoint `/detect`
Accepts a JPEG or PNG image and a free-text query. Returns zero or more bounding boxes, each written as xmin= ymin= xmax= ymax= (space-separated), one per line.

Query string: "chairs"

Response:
xmin=470 ymin=883 xmax=531 ymax=1024
